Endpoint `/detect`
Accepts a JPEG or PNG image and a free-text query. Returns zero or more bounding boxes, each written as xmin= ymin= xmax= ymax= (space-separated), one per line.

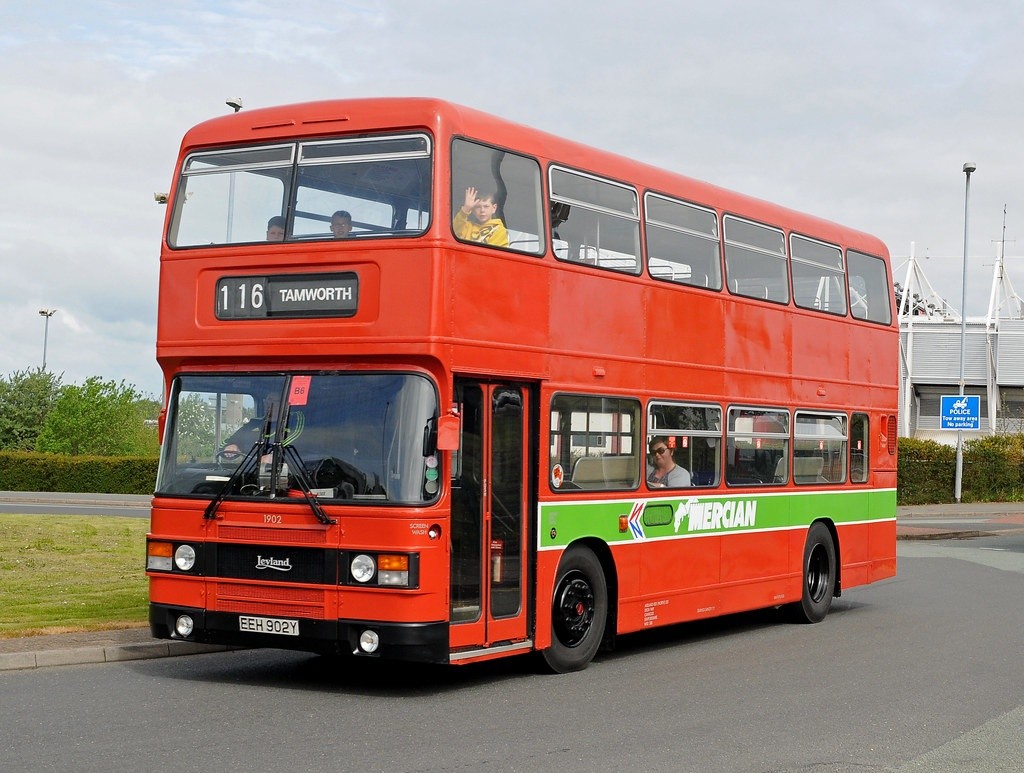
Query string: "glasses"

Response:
xmin=335 ymin=222 xmax=346 ymax=228
xmin=650 ymin=447 xmax=669 ymax=457
xmin=263 ymin=398 xmax=281 ymax=407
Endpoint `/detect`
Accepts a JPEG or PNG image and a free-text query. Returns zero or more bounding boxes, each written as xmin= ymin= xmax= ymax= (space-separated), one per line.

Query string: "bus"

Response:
xmin=135 ymin=94 xmax=902 ymax=679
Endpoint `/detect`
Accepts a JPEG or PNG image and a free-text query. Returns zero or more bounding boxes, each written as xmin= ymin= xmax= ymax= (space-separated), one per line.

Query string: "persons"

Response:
xmin=647 ymin=436 xmax=692 ymax=487
xmin=218 ymin=390 xmax=305 ymax=460
xmin=329 ymin=211 xmax=352 ymax=238
xmin=266 ymin=214 xmax=290 ymax=241
xmin=453 ymin=186 xmax=508 ymax=248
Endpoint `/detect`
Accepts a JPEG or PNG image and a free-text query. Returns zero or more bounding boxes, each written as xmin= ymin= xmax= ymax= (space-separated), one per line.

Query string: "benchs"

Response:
xmin=290 ymin=228 xmax=867 ymax=321
xmin=570 ymin=457 xmax=829 ymax=489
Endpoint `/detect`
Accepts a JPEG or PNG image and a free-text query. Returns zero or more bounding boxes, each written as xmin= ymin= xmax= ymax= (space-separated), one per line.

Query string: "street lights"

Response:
xmin=36 ymin=308 xmax=56 ymax=376
xmin=953 ymin=161 xmax=977 ymax=505
xmin=211 ymin=94 xmax=242 ymax=466
